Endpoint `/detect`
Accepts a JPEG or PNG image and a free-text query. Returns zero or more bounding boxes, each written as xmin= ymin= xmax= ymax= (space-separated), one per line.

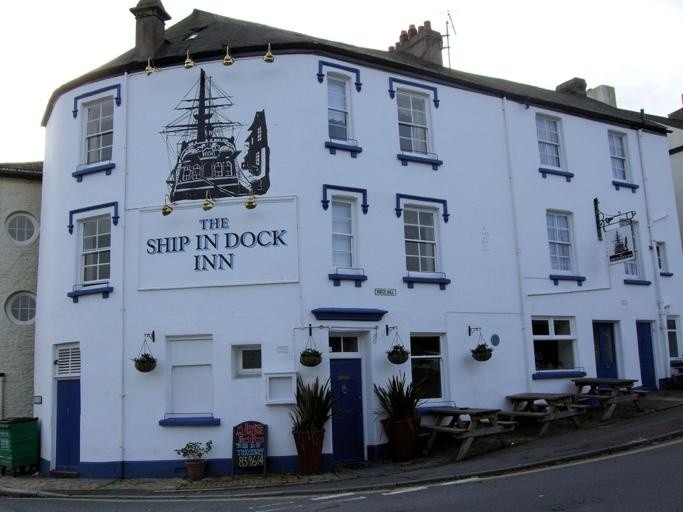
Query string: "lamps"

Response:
xmin=145 ymin=42 xmax=277 ymax=76
xmin=161 ymin=187 xmax=257 ymax=217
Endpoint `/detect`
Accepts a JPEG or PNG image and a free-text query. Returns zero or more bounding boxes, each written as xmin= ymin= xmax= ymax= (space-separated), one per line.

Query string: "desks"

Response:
xmin=570 ymin=377 xmax=643 ymax=423
xmin=416 ymin=404 xmax=511 ymax=463
xmin=506 ymin=392 xmax=583 ymax=439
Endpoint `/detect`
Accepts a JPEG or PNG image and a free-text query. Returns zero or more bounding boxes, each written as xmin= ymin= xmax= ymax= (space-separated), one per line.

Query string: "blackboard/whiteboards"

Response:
xmin=232 ymin=420 xmax=268 ymax=475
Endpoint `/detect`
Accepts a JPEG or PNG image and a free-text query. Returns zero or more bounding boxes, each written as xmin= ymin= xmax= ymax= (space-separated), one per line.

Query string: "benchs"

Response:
xmin=497 ymin=403 xmax=594 ymax=436
xmin=581 ymin=387 xmax=649 ymax=426
xmin=415 ymin=420 xmax=518 ymax=460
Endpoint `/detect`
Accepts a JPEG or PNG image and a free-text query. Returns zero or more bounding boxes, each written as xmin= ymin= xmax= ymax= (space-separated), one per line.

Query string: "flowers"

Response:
xmin=129 ymin=337 xmax=157 ymax=373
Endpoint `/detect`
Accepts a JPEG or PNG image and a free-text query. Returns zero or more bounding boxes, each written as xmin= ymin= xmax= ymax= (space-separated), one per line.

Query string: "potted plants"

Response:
xmin=171 ymin=435 xmax=213 ymax=482
xmin=368 ymin=324 xmax=432 ymax=463
xmin=466 ymin=324 xmax=494 ymax=363
xmin=290 ymin=323 xmax=356 ymax=477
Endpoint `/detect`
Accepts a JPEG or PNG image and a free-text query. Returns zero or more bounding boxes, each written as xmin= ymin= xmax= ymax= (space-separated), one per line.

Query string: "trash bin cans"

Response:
xmin=0 ymin=418 xmax=41 ymax=477
